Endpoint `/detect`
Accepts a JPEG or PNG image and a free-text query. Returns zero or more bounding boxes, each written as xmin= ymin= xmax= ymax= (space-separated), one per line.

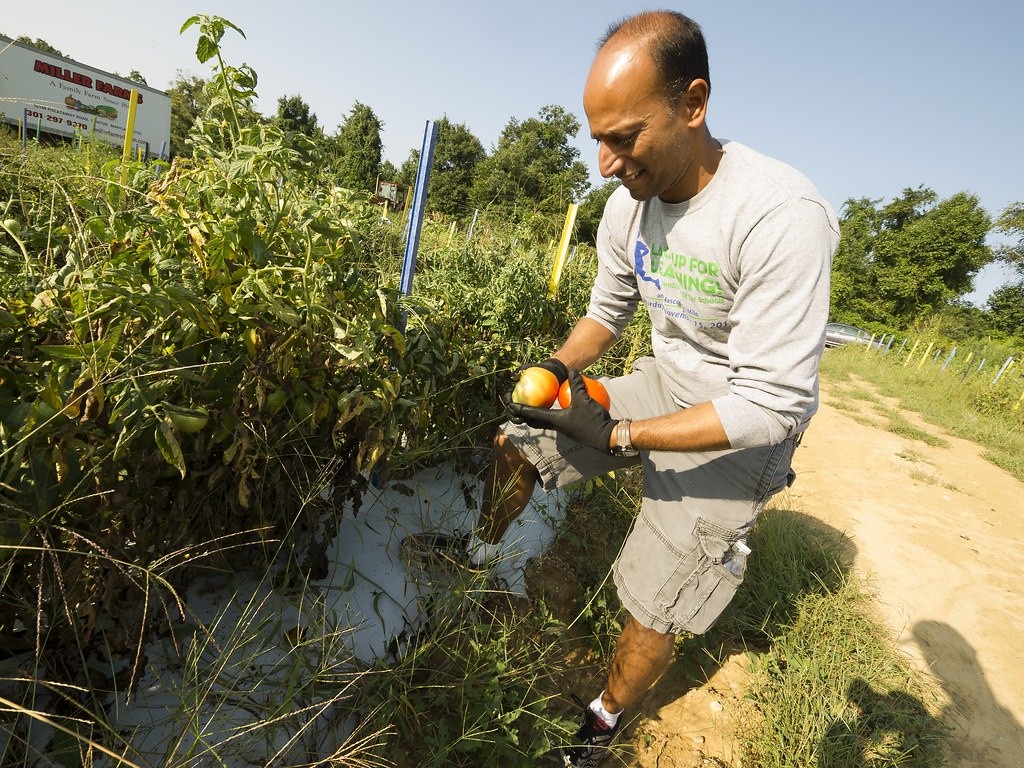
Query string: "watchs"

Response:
xmin=610 ymin=417 xmax=640 ymax=458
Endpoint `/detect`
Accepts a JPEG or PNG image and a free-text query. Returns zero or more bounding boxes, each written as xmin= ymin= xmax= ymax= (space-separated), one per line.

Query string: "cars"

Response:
xmin=824 ymin=322 xmax=887 ymax=351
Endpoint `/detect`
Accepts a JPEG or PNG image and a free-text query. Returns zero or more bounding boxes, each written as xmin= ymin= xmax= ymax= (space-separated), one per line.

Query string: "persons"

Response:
xmin=401 ymin=8 xmax=841 ymax=768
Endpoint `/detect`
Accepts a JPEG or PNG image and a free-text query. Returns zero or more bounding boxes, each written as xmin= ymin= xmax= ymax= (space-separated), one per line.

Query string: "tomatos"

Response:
xmin=559 ymin=374 xmax=610 ymax=412
xmin=32 ymin=400 xmax=69 ymax=425
xmin=512 ymin=367 xmax=559 ymax=410
xmin=169 ymin=404 xmax=209 ymax=433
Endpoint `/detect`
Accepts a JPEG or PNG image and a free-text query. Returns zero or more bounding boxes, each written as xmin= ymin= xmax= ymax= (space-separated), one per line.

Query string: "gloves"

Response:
xmin=508 ymin=358 xmax=569 ymax=424
xmin=509 ymin=367 xmax=619 ymax=456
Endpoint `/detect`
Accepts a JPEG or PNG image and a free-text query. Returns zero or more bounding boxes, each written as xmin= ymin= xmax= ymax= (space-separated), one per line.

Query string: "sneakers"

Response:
xmin=557 ymin=692 xmax=623 ymax=768
xmin=399 ymin=529 xmax=499 ymax=573
